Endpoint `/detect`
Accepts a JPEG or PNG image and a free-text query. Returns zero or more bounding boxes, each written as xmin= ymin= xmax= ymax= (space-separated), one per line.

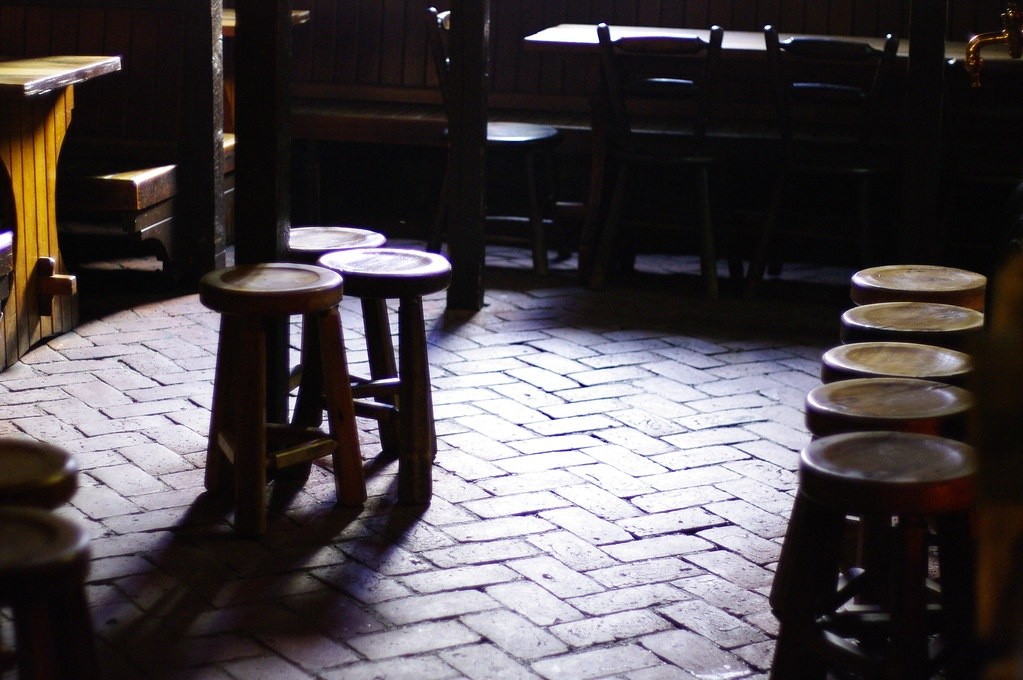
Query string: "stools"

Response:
xmin=1 ymin=434 xmax=107 ymax=679
xmin=766 ymin=259 xmax=991 ymax=680
xmin=197 ymin=227 xmax=457 ymax=544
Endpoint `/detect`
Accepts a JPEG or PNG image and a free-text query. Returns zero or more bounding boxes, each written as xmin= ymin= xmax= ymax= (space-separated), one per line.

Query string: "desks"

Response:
xmin=527 ymin=23 xmax=1023 ymax=297
xmin=0 ymin=52 xmax=121 ymax=367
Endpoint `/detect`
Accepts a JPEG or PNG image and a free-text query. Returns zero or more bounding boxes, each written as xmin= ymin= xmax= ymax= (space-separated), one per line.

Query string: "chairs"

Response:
xmin=422 ymin=6 xmax=1022 ymax=294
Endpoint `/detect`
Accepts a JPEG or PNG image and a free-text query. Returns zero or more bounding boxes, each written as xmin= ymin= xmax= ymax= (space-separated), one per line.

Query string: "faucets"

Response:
xmin=964 ymin=1 xmax=1023 ymax=74
